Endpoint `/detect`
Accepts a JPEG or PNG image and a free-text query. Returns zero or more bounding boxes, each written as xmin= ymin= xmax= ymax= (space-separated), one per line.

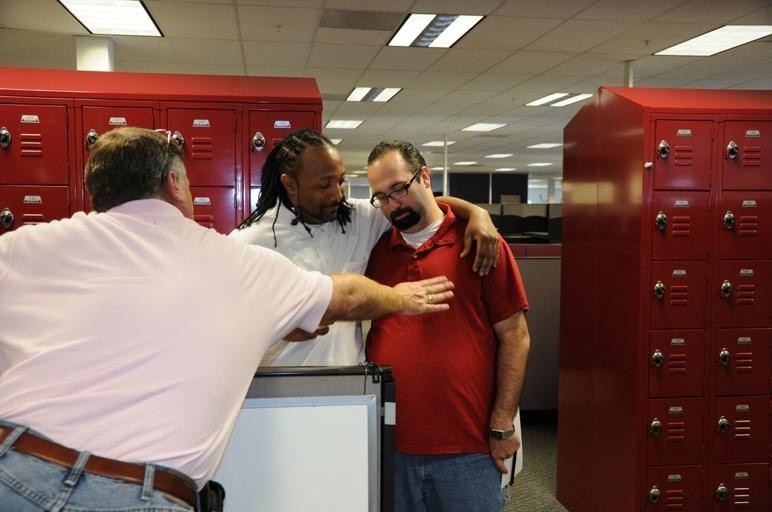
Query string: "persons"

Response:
xmin=223 ymin=131 xmax=501 ymax=368
xmin=366 ymin=138 xmax=532 ymax=511
xmin=1 ymin=127 xmax=455 ymax=512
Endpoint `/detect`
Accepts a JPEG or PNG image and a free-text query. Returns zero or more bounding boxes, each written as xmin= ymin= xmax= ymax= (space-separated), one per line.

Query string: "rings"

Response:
xmin=429 ymin=294 xmax=432 ymax=303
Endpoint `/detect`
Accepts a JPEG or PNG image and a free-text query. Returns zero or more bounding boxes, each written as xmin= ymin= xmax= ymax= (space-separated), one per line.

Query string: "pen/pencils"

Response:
xmin=509 ymin=451 xmax=517 ymax=486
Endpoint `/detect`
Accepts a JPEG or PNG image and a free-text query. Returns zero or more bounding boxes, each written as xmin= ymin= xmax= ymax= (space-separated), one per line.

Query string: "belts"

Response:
xmin=0 ymin=426 xmax=196 ymax=508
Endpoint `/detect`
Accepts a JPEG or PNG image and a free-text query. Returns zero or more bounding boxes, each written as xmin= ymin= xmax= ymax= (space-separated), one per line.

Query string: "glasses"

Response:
xmin=369 ymin=169 xmax=419 ymax=209
xmin=152 ymin=128 xmax=171 ymax=183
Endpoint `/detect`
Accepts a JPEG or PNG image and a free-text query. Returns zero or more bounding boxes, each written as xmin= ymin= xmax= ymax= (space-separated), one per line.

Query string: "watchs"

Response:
xmin=488 ymin=425 xmax=516 ymax=441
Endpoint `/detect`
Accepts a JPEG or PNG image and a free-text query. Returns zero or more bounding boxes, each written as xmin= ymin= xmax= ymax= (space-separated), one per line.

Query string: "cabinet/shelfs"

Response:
xmin=554 ymin=83 xmax=771 ymax=511
xmin=0 ymin=66 xmax=325 ymax=237
